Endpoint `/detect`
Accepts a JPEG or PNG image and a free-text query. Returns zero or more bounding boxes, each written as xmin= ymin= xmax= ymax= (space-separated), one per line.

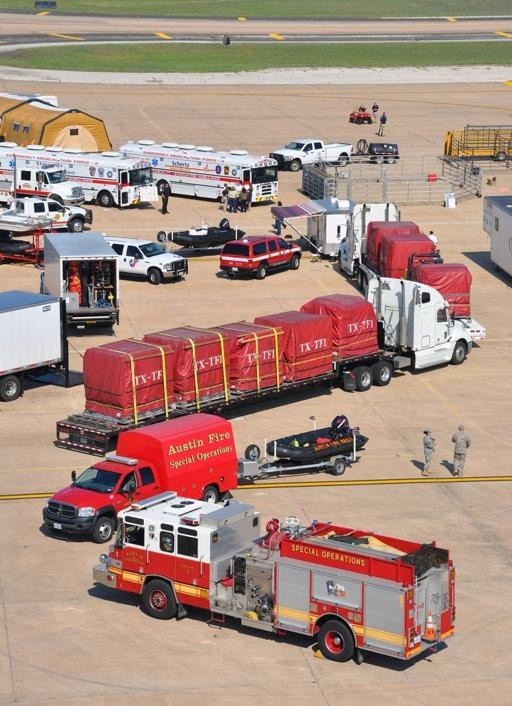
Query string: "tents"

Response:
xmin=1 ymin=98 xmax=114 ymax=152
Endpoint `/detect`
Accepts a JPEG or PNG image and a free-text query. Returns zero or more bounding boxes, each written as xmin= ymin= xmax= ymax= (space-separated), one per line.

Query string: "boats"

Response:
xmin=167 ymin=217 xmax=246 ymax=249
xmin=267 ymin=414 xmax=371 ymax=462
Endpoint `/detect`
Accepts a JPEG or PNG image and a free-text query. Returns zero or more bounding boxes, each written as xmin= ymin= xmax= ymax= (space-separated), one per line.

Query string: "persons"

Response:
xmin=274 ymin=201 xmax=286 ymax=229
xmin=372 ymin=102 xmax=379 ymax=122
xmin=358 ymin=104 xmax=367 ymax=112
xmin=221 ymin=182 xmax=254 ymax=212
xmin=376 ymin=111 xmax=387 ymax=136
xmin=160 ymin=183 xmax=171 ymax=215
xmin=422 ymin=427 xmax=437 ymax=476
xmin=428 ymin=231 xmax=438 ymax=246
xmin=450 ymin=424 xmax=473 ymax=477
xmin=275 ymin=214 xmax=283 ymax=235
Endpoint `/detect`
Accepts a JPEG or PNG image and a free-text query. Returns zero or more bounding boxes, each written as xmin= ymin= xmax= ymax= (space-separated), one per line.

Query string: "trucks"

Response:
xmin=1 ymin=289 xmax=68 ymax=402
xmin=41 ymin=413 xmax=240 ymax=543
xmin=40 ymin=232 xmax=121 ymax=329
xmin=1 ymin=155 xmax=87 ymax=208
xmin=338 ymin=201 xmax=486 ymax=343
xmin=53 ymin=277 xmax=474 ymax=455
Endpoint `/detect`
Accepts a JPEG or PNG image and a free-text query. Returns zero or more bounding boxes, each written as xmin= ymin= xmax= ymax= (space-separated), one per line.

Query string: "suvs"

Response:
xmin=105 ymin=235 xmax=190 ymax=283
xmin=219 ymin=235 xmax=303 ymax=278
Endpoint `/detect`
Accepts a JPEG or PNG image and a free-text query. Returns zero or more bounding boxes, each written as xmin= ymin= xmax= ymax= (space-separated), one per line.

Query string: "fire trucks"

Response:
xmin=96 ymin=493 xmax=456 ymax=668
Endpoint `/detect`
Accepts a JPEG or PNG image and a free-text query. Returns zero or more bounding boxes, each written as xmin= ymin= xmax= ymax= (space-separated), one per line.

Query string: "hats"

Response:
xmin=458 ymin=423 xmax=465 ymax=430
xmin=425 ymin=427 xmax=433 ymax=433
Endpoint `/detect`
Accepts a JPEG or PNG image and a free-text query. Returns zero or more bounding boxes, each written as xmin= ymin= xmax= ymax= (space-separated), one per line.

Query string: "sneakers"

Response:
xmin=452 ymin=468 xmax=464 ymax=477
xmin=421 ymin=468 xmax=433 ymax=476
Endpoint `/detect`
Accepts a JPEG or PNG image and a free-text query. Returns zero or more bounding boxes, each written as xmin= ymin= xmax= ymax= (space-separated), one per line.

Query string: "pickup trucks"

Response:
xmin=272 ymin=139 xmax=351 ymax=171
xmin=1 ymin=197 xmax=94 ymax=232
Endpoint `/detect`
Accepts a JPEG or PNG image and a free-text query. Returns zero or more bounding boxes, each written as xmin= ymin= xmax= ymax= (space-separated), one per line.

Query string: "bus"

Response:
xmin=120 ymin=141 xmax=283 ymax=207
xmin=0 ymin=141 xmax=159 ymax=209
